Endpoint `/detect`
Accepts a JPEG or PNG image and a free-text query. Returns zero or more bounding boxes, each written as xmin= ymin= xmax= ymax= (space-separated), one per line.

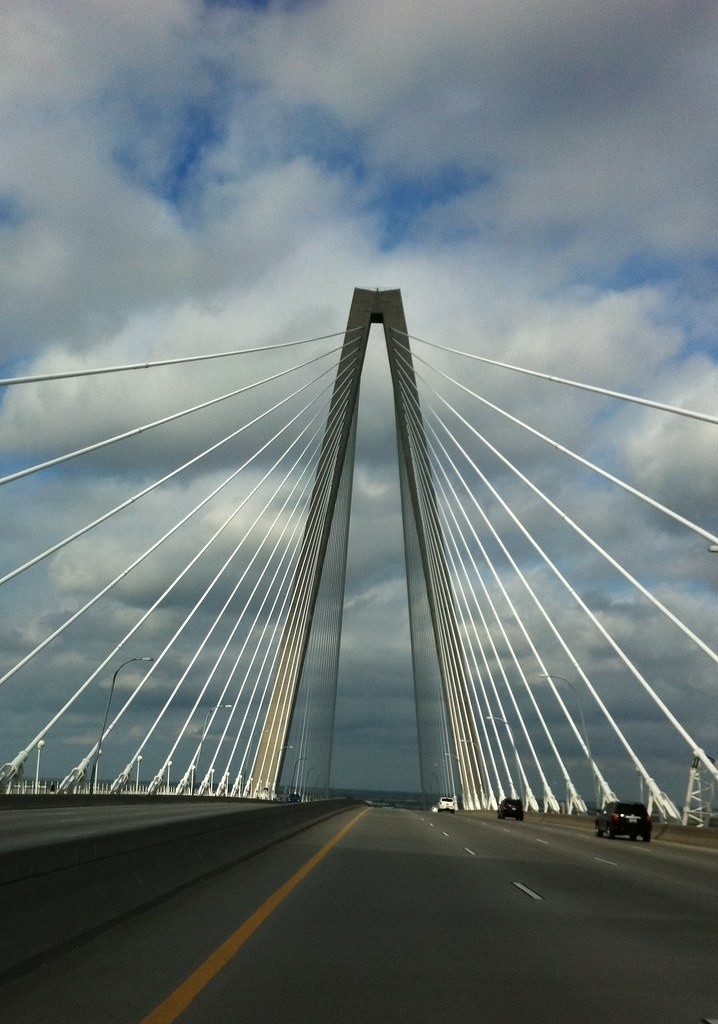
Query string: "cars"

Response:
xmin=497 ymin=798 xmax=524 ymax=820
xmin=288 ymin=793 xmax=300 ymax=801
xmin=438 ymin=797 xmax=456 ymax=813
xmin=596 ymin=801 xmax=652 ymax=840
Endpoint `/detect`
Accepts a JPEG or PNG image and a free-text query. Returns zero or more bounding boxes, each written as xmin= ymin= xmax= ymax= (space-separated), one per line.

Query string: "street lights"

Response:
xmin=540 ymin=674 xmax=598 ymax=799
xmin=90 ymin=658 xmax=153 ymax=796
xmin=459 ymin=739 xmax=489 ymax=803
xmin=262 ymin=746 xmax=295 ymax=796
xmin=487 ymin=717 xmax=523 ymax=794
xmin=199 ymin=705 xmax=232 ymax=755
